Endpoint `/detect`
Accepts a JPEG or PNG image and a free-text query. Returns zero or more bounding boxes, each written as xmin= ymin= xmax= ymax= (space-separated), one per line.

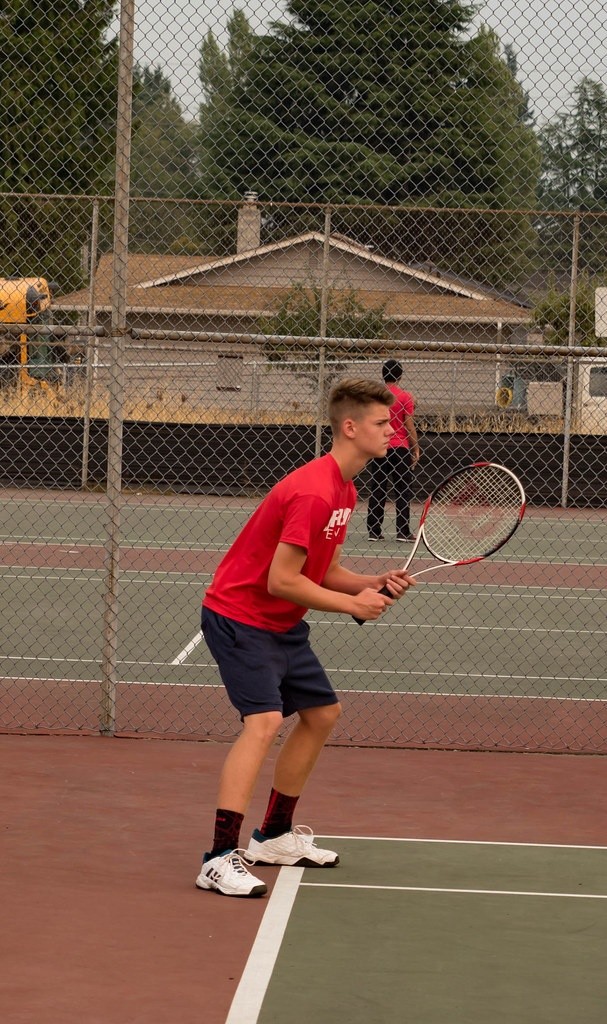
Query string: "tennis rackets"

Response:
xmin=349 ymin=460 xmax=529 ymax=626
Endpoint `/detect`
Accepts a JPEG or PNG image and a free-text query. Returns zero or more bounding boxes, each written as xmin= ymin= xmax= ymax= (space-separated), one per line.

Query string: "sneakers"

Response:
xmin=368 ymin=533 xmax=385 ymax=541
xmin=195 ymin=849 xmax=268 ymax=897
xmin=396 ymin=533 xmax=421 ymax=542
xmin=244 ymin=825 xmax=340 ymax=868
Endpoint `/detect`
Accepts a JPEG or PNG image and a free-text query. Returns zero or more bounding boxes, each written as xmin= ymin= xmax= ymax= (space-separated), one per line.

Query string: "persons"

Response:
xmin=199 ymin=380 xmax=417 ymax=896
xmin=367 ymin=360 xmax=419 ymax=543
